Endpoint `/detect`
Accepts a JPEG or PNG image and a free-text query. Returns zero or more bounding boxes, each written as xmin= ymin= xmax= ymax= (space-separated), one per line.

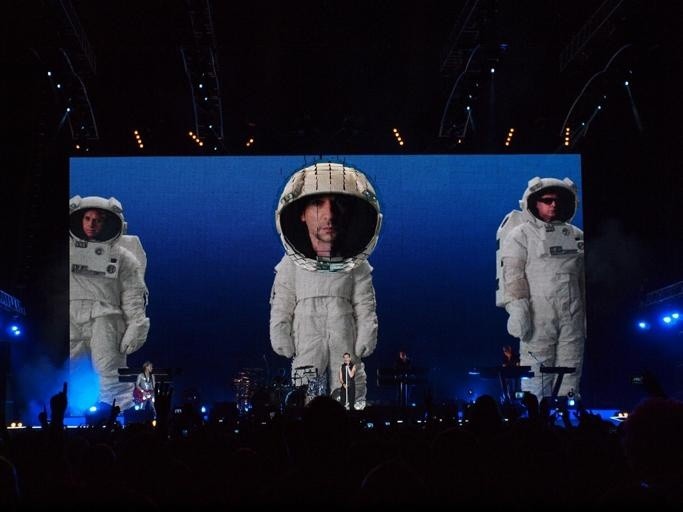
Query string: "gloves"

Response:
xmin=270 ymin=317 xmax=294 ymax=358
xmin=505 ymin=298 xmax=531 ymax=341
xmin=355 ymin=325 xmax=377 ymax=357
xmin=120 ymin=317 xmax=150 ymax=355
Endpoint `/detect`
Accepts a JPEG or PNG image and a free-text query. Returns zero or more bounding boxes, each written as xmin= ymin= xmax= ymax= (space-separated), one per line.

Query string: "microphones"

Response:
xmin=345 ymin=362 xmax=348 ymax=368
xmin=527 ymin=350 xmax=533 ymax=356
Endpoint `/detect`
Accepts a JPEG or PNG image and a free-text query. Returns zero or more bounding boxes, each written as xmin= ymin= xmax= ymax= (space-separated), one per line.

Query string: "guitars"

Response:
xmin=133 ymin=387 xmax=169 ymax=404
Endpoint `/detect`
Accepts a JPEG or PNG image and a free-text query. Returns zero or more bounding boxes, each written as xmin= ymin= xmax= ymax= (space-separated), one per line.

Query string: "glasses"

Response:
xmin=536 ymin=196 xmax=567 ymax=205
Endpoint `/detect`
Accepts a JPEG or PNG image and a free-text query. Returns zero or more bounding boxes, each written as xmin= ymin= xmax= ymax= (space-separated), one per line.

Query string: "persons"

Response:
xmin=499 ymin=345 xmax=516 ymax=403
xmin=233 ymin=372 xmax=252 ymax=417
xmin=394 ymin=351 xmax=413 ymax=404
xmin=68 ymin=195 xmax=153 ymax=410
xmin=272 ymin=368 xmax=289 ymax=412
xmin=1 ymin=380 xmax=682 ymax=510
xmin=135 ymin=360 xmax=158 ymax=422
xmin=341 ymin=353 xmax=356 ymax=411
xmin=497 ymin=177 xmax=587 ymax=404
xmin=269 ymin=162 xmax=379 ymax=410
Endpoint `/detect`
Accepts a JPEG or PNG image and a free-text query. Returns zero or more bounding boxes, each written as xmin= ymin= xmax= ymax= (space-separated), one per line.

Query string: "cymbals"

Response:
xmin=291 ymin=376 xmax=300 ymax=379
xmin=305 ymin=372 xmax=314 ymax=373
xmin=294 ymin=365 xmax=312 ymax=370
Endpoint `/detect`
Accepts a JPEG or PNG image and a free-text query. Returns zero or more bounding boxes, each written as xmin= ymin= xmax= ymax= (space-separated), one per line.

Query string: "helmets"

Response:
xmin=68 ymin=195 xmax=127 ymax=242
xmin=519 ymin=176 xmax=579 ymax=227
xmin=275 ymin=162 xmax=383 ymax=272
